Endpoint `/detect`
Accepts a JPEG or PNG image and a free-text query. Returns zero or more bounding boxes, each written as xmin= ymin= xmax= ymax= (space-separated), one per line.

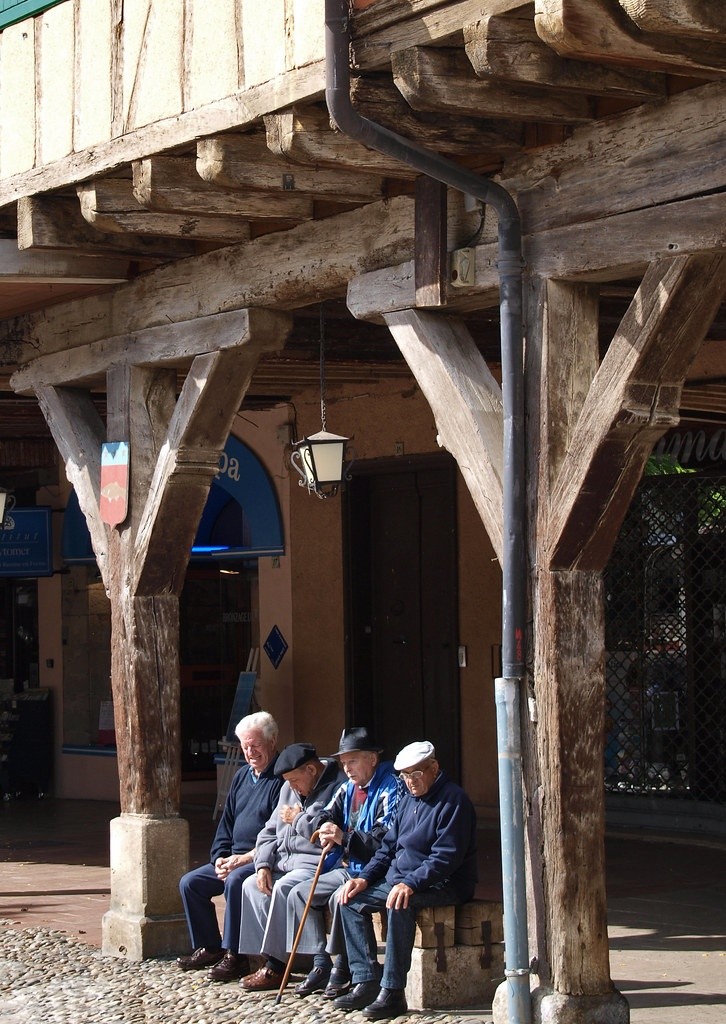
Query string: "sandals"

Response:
xmin=322 ymin=968 xmax=351 ymax=1000
xmin=294 ymin=966 xmax=331 ymax=995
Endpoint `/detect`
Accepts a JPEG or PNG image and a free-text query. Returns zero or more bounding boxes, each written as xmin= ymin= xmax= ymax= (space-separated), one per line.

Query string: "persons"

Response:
xmin=240 ymin=742 xmax=346 ymax=988
xmin=286 ymin=724 xmax=407 ymax=999
xmin=334 ymin=741 xmax=477 ymax=1017
xmin=176 ymin=710 xmax=288 ymax=979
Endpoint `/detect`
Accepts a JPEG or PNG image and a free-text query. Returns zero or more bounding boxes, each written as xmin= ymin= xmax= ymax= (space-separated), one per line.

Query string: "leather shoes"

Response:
xmin=362 ymin=987 xmax=407 ymax=1017
xmin=239 ymin=966 xmax=289 ymax=989
xmin=207 ymin=953 xmax=249 ymax=980
xmin=333 ymin=981 xmax=379 ymax=1008
xmin=176 ymin=947 xmax=226 ymax=969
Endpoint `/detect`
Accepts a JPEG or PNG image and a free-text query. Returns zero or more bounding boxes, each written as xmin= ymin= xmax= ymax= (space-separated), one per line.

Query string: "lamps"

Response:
xmin=290 ymin=304 xmax=358 ymax=501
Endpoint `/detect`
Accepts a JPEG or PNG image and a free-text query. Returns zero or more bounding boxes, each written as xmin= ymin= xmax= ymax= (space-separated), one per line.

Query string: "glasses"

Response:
xmin=398 ymin=762 xmax=434 ymax=780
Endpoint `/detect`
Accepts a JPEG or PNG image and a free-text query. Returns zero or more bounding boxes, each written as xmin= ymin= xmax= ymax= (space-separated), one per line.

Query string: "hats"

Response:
xmin=273 ymin=743 xmax=316 ymax=776
xmin=329 ymin=727 xmax=385 ymax=758
xmin=392 ymin=740 xmax=435 ymax=771
